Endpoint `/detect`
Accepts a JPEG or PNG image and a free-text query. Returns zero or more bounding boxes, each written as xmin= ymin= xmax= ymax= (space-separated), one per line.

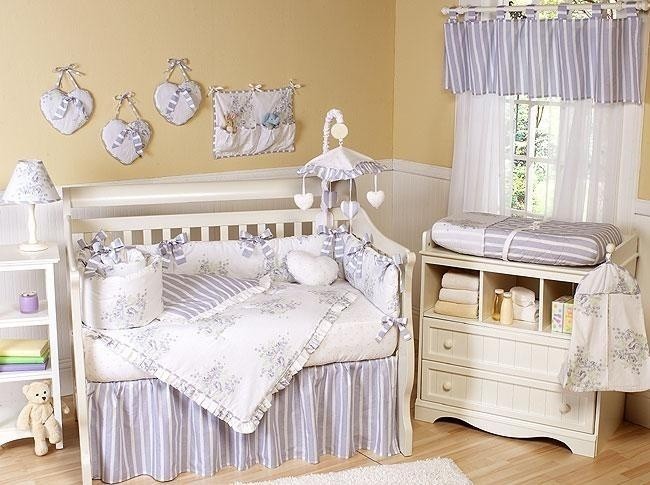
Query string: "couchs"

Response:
xmin=63 ymin=176 xmax=417 ymax=485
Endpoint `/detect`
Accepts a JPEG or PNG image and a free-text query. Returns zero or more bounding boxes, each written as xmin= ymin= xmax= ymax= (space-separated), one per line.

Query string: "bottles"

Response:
xmin=491 ymin=289 xmax=513 ymax=327
xmin=20 ymin=291 xmax=38 ymax=314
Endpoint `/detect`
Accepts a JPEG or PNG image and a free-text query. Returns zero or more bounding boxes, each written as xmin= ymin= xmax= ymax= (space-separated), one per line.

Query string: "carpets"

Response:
xmin=230 ymin=457 xmax=475 ymax=485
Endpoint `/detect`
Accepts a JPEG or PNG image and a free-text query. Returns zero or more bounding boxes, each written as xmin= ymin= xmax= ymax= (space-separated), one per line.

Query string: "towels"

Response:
xmin=433 ymin=270 xmax=480 ymax=319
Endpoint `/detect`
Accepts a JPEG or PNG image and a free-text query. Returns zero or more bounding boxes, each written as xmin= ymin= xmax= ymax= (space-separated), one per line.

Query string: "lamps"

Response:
xmin=1 ymin=159 xmax=62 ymax=252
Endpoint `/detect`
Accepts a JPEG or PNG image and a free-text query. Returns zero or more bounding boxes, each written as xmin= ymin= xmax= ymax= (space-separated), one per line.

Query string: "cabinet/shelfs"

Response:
xmin=0 ymin=243 xmax=63 ymax=451
xmin=414 ymin=229 xmax=639 ymax=458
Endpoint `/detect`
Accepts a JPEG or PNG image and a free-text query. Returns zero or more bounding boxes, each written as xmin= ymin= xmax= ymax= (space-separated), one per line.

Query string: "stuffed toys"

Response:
xmin=16 ymin=380 xmax=70 ymax=456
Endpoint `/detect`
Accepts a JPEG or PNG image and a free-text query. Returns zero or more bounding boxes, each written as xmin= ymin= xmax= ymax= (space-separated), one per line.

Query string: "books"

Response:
xmin=0 ymin=358 xmax=49 ymax=372
xmin=0 ymin=350 xmax=50 ymax=365
xmin=0 ymin=339 xmax=49 ymax=357
